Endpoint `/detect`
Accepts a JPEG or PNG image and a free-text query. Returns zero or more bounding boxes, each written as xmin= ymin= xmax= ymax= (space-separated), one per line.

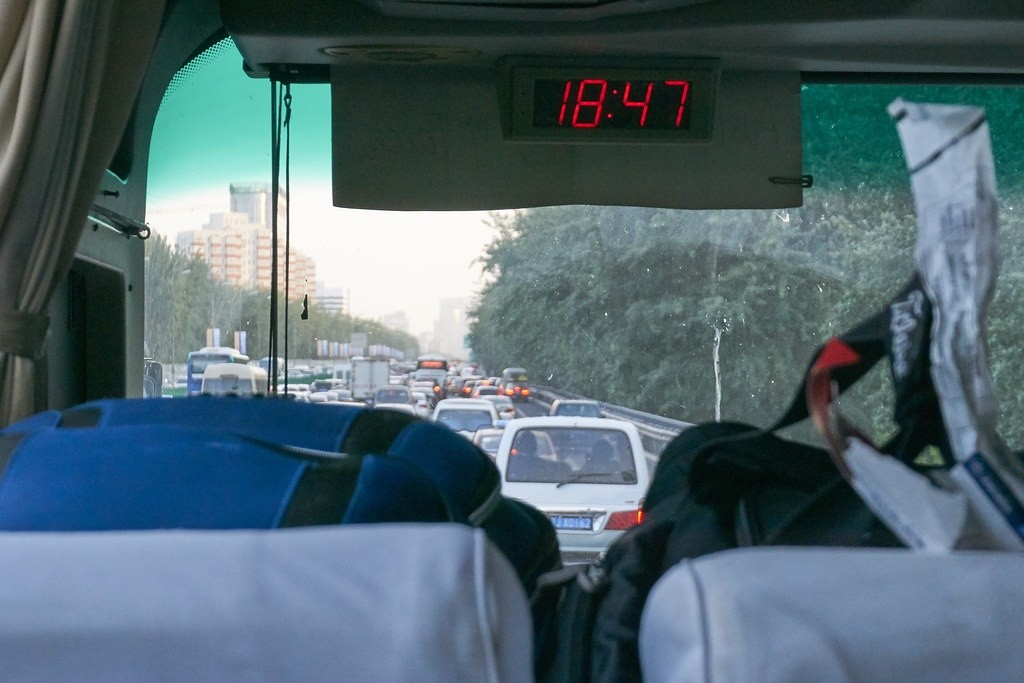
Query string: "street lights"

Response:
xmin=211 ymin=273 xmax=270 ymax=355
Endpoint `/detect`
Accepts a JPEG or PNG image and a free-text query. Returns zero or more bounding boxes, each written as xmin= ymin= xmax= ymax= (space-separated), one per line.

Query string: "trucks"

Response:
xmin=351 ymin=356 xmax=390 ymax=402
xmin=502 ymin=368 xmax=528 ymax=390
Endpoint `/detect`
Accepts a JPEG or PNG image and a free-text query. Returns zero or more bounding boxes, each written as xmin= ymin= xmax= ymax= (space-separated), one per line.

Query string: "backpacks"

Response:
xmin=529 ymin=271 xmax=957 ymax=683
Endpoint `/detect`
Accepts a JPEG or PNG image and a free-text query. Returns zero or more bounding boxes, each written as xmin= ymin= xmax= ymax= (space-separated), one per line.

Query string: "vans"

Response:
xmin=494 ymin=416 xmax=650 ymax=563
xmin=433 ymin=398 xmax=498 ymax=443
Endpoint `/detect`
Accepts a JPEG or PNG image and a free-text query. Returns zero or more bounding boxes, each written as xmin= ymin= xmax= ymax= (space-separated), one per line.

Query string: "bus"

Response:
xmin=259 ymin=356 xmax=285 ymax=376
xmin=143 ymin=342 xmax=174 ymax=399
xmin=415 ymin=357 xmax=449 ymax=385
xmin=201 ymin=365 xmax=269 ymax=397
xmin=188 ymin=347 xmax=250 ymax=400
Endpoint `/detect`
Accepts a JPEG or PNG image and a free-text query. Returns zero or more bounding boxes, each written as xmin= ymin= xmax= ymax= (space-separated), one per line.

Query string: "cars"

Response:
xmin=271 ymin=362 xmax=528 ymax=419
xmin=549 ymin=398 xmax=606 ymax=443
xmin=174 ymin=377 xmax=187 ymax=390
xmin=473 ymin=428 xmax=559 ymax=463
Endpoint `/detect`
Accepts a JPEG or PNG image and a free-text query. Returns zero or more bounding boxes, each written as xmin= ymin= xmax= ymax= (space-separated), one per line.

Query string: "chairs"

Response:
xmin=635 ymin=547 xmax=1023 ymax=682
xmin=0 ymin=401 xmax=562 ymax=683
